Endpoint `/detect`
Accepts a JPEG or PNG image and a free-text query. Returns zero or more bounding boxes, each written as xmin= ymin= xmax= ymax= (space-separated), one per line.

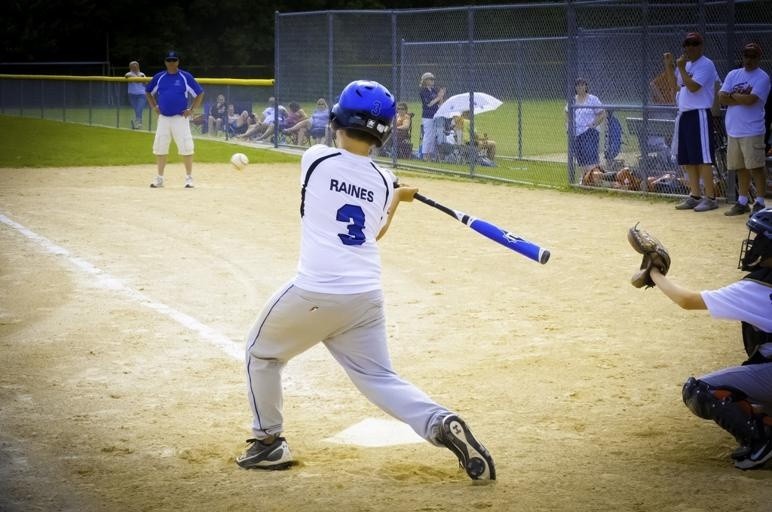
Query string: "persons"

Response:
xmin=207 ymin=94 xmax=337 ymax=147
xmin=419 ymin=72 xmax=447 ymax=163
xmin=564 ymin=31 xmax=771 ymax=218
xmin=380 ymin=102 xmax=411 ymax=157
xmin=125 ymin=61 xmax=149 ymax=129
xmin=627 ymin=206 xmax=772 ymax=471
xmin=143 ymin=50 xmax=205 ymax=188
xmin=461 ymin=111 xmax=496 ymax=167
xmin=234 ymin=79 xmax=495 ymax=485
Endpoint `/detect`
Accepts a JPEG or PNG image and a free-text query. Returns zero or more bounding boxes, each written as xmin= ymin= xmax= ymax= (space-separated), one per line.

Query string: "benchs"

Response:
xmin=625 ymin=118 xmax=723 ymax=179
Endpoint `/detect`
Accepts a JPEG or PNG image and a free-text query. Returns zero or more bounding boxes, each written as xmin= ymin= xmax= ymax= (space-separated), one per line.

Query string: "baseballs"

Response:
xmin=230 ymin=153 xmax=248 ymax=171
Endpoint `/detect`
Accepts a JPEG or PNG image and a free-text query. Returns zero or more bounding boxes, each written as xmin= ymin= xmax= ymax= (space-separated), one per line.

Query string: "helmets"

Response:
xmin=329 ymin=80 xmax=398 ymax=147
xmin=738 ymin=206 xmax=772 ymax=271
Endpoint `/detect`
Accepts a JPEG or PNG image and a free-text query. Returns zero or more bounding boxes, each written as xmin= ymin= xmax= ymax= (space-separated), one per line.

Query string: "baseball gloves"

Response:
xmin=627 ymin=222 xmax=671 ymax=290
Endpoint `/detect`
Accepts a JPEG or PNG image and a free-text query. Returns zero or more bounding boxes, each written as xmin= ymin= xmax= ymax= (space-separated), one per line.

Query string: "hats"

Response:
xmin=164 ymin=52 xmax=178 ymax=60
xmin=682 ymin=32 xmax=704 ymax=48
xmin=421 ymin=72 xmax=437 ymax=82
xmin=742 ymin=42 xmax=763 ymax=56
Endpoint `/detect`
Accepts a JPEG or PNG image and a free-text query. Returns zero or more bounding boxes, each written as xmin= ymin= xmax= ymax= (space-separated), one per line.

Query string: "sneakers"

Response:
xmin=235 ymin=437 xmax=294 ymax=471
xmin=694 ymin=197 xmax=719 ymax=212
xmin=749 ymin=201 xmax=765 ymax=218
xmin=731 ymin=437 xmax=772 ymax=471
xmin=150 ymin=178 xmax=164 ymax=187
xmin=724 ymin=200 xmax=750 ymax=216
xmin=184 ymin=178 xmax=194 ymax=188
xmin=675 ymin=196 xmax=702 ymax=209
xmin=440 ymin=414 xmax=496 ymax=480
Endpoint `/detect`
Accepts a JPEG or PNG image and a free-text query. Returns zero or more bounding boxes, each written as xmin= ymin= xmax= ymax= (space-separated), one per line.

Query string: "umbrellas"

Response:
xmin=433 ymin=92 xmax=504 ymax=120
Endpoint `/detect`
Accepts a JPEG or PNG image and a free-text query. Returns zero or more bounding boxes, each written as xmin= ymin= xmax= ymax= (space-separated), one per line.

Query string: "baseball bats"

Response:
xmin=393 ymin=182 xmax=550 ymax=264
